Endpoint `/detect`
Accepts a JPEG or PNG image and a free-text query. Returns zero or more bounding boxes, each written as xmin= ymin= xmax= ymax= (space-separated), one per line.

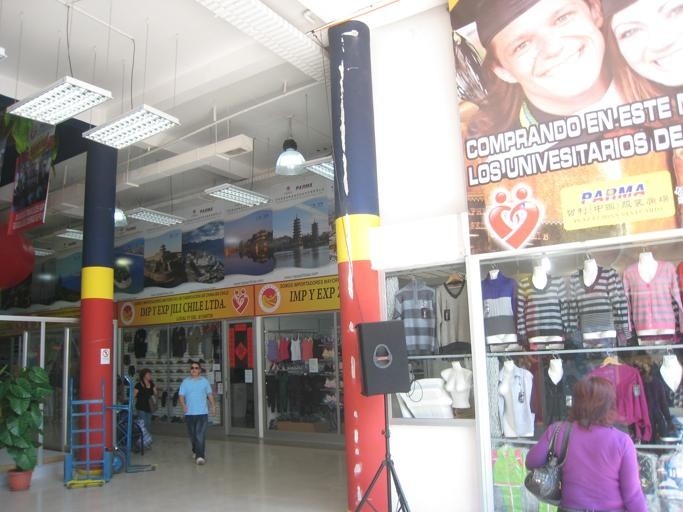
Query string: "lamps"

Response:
xmin=32 ymin=247 xmax=56 ymax=257
xmin=114 ymin=192 xmax=127 ymax=227
xmin=124 ymin=174 xmax=187 ymax=227
xmin=54 ymin=217 xmax=83 ymax=240
xmin=274 ymin=112 xmax=308 ymax=177
xmin=82 ymin=17 xmax=182 ymax=149
xmin=203 ymin=136 xmax=272 ymax=208
xmin=300 ymin=92 xmax=334 ymax=182
xmin=5 ymin=0 xmax=113 ymax=124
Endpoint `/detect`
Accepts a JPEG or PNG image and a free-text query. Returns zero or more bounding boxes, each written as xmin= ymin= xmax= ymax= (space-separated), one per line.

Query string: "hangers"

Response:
xmin=405 ymin=264 xmax=464 ymax=284
xmin=600 ymin=350 xmax=625 ymax=369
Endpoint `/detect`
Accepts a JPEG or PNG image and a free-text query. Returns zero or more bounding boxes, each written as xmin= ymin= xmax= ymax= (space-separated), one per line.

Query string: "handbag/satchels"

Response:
xmin=148 ymin=395 xmax=157 ymax=413
xmin=523 ymin=419 xmax=572 ymax=508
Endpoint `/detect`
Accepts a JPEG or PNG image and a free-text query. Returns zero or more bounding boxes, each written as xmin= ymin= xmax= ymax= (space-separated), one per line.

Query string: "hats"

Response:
xmin=450 ymin=0 xmax=542 ymax=49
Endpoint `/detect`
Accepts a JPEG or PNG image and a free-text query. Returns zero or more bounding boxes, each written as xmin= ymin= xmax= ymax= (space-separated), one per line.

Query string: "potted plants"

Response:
xmin=0 ymin=363 xmax=55 ymax=491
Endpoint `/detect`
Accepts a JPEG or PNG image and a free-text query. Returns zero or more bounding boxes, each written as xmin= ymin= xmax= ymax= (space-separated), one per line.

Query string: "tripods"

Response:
xmin=355 ymin=394 xmax=410 ymax=512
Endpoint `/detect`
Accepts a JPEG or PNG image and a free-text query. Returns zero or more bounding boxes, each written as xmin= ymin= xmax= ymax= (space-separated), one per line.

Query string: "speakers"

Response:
xmin=358 ymin=320 xmax=411 ymax=396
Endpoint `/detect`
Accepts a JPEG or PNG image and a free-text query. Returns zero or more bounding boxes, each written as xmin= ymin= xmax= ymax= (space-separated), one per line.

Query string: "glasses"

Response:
xmin=189 ymin=367 xmax=198 ymax=370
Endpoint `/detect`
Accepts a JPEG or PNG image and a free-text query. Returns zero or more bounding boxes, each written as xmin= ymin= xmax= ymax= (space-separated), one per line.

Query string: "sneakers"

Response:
xmin=192 ymin=450 xmax=207 ymax=466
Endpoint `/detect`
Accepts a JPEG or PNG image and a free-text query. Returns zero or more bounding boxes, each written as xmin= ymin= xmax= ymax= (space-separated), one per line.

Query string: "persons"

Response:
xmin=525 ymin=376 xmax=649 ymax=512
xmin=481 ymin=251 xmax=683 ymax=442
xmin=440 ymin=361 xmax=472 ymax=409
xmin=178 ymin=362 xmax=217 ymax=465
xmin=133 ymin=368 xmax=158 ymax=433
